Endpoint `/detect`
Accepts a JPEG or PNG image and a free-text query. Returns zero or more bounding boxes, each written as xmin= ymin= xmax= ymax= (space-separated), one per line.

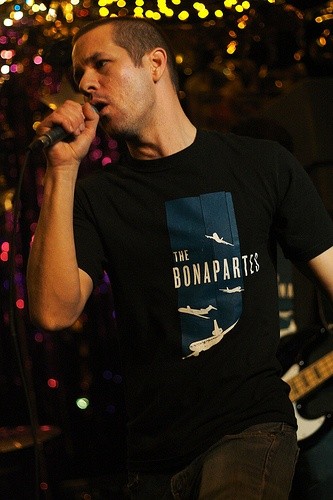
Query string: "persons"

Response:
xmin=26 ymin=17 xmax=332 ymax=499
xmin=273 ymin=239 xmax=331 ymax=500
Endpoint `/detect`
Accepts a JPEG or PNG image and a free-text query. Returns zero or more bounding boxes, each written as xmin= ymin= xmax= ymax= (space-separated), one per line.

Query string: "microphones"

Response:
xmin=29 ymin=105 xmax=98 ymax=156
